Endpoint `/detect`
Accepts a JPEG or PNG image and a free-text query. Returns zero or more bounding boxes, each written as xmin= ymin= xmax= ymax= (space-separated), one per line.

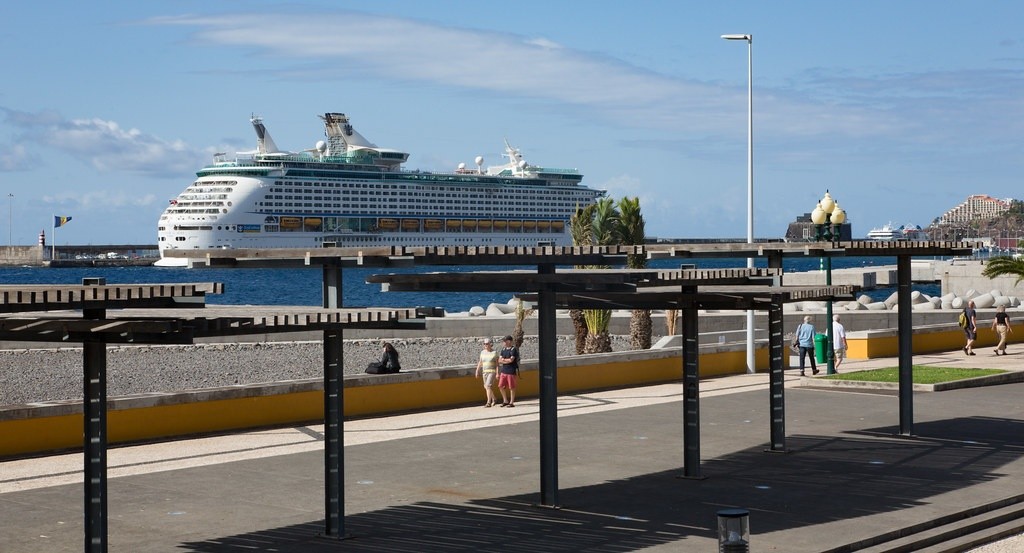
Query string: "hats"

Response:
xmin=501 ymin=334 xmax=513 ymax=340
xmin=483 ymin=338 xmax=492 ymax=343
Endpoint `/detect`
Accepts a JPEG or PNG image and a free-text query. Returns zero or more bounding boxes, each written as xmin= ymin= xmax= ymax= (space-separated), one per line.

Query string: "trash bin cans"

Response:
xmin=814 ymin=333 xmax=828 ymax=364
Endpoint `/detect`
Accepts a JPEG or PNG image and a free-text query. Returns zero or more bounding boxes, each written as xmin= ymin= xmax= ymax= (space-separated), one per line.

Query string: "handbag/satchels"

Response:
xmin=365 ymin=362 xmax=382 ymax=373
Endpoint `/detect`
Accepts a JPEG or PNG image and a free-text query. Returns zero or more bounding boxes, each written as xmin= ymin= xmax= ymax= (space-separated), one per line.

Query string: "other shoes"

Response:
xmin=484 ymin=402 xmax=491 ymax=407
xmin=813 ymin=369 xmax=819 ymax=374
xmin=801 ymin=372 xmax=805 ymax=375
xmin=500 ymin=401 xmax=509 ymax=406
xmin=1002 ymin=352 xmax=1007 ymax=354
xmin=963 ymin=347 xmax=969 ymax=354
xmin=506 ymin=403 xmax=514 ymax=407
xmin=994 ymin=349 xmax=999 ymax=354
xmin=970 ymin=351 xmax=976 ymax=354
xmin=492 ymin=400 xmax=497 ymax=405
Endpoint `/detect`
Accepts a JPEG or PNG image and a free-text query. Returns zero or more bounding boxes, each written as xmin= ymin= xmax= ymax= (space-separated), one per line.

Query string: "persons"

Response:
xmin=990 ymin=305 xmax=1013 ymax=356
xmin=475 ymin=337 xmax=500 ymax=408
xmin=795 ymin=315 xmax=819 ymax=376
xmin=825 ymin=314 xmax=848 ymax=372
xmin=497 ymin=334 xmax=517 ymax=407
xmin=374 ymin=343 xmax=401 ymax=373
xmin=962 ymin=300 xmax=978 ymax=356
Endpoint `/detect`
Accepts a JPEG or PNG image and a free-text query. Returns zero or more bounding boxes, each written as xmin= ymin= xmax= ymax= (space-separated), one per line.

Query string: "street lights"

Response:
xmin=809 ymin=188 xmax=846 ymax=376
xmin=722 ymin=33 xmax=756 ymax=374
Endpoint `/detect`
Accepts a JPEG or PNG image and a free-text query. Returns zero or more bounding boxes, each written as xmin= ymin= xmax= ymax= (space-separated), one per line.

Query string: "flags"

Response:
xmin=55 ymin=215 xmax=72 ymax=228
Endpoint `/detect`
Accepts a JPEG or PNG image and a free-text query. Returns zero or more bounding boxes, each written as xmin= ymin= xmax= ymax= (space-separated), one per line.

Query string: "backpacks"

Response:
xmin=959 ymin=307 xmax=967 ymax=328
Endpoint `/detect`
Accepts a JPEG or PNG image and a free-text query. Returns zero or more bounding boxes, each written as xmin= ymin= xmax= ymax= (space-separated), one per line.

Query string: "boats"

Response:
xmin=509 ymin=220 xmax=522 ymax=228
xmin=494 ymin=220 xmax=508 ymax=228
xmin=523 ymin=220 xmax=536 ymax=228
xmin=478 ymin=220 xmax=492 ymax=228
xmin=424 ymin=218 xmax=444 ymax=230
xmin=280 ymin=217 xmax=302 ymax=228
xmin=446 ymin=219 xmax=460 ymax=228
xmin=537 ymin=221 xmax=550 ymax=229
xmin=402 ymin=218 xmax=420 ymax=230
xmin=378 ymin=217 xmax=399 ymax=229
xmin=551 ymin=221 xmax=564 ymax=229
xmin=463 ymin=219 xmax=477 ymax=228
xmin=865 ymin=220 xmax=904 ymax=243
xmin=304 ymin=217 xmax=323 ymax=228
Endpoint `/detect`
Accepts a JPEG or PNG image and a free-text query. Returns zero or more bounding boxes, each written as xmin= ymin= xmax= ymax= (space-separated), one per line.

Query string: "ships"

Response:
xmin=152 ymin=112 xmax=609 ymax=269
